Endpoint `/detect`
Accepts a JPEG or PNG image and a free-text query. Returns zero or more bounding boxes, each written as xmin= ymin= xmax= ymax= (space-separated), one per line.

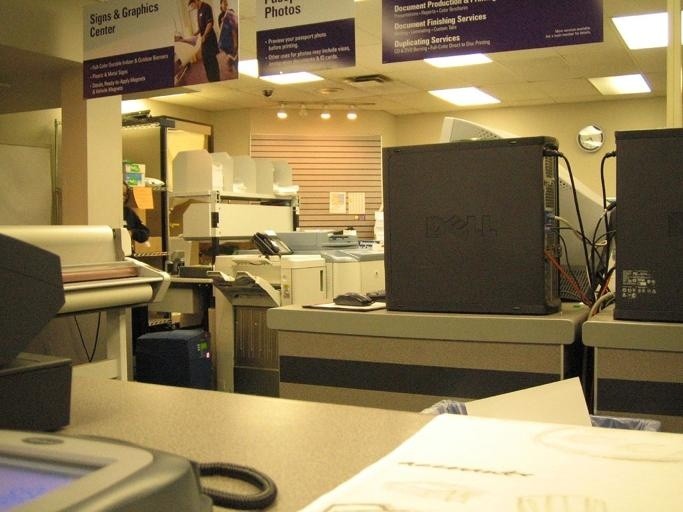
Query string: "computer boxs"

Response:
xmin=614 ymin=127 xmax=683 ymax=324
xmin=136 ymin=329 xmax=216 ymax=390
xmin=382 ymin=136 xmax=561 ymax=315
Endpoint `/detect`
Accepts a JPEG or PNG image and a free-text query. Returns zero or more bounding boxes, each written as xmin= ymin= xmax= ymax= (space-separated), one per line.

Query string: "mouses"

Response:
xmin=332 ymin=292 xmax=372 ymax=306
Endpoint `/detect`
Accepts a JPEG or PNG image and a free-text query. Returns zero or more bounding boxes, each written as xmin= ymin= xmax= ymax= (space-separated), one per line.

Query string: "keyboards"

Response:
xmin=366 ymin=291 xmax=385 ymax=302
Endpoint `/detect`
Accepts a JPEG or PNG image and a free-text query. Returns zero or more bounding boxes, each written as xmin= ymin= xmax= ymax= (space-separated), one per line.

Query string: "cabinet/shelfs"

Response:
xmin=164 ymin=190 xmax=301 ymax=280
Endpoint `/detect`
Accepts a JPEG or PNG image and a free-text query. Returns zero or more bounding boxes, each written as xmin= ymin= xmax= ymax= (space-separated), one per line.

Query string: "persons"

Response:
xmin=187 ymin=0 xmax=221 ymax=82
xmin=217 ymin=0 xmax=238 ymax=70
xmin=123 ymin=180 xmax=151 ymax=243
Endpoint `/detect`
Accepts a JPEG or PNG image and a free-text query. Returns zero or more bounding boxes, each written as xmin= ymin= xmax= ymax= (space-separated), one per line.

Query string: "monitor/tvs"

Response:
xmin=441 ymin=116 xmax=613 ymax=303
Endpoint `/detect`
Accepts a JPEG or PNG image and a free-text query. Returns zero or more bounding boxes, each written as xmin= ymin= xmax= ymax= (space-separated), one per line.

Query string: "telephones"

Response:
xmin=251 ymin=232 xmax=294 ymax=258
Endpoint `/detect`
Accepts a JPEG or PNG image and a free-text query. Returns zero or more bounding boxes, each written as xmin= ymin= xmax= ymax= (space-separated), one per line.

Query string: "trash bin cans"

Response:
xmin=421 ymin=400 xmax=661 ymax=432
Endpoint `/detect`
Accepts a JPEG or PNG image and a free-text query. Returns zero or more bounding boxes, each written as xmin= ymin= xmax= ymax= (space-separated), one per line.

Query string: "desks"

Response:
xmin=71 ymin=376 xmax=441 ymax=512
xmin=266 ymin=303 xmax=590 ymax=413
xmin=583 ymin=304 xmax=681 ymax=415
